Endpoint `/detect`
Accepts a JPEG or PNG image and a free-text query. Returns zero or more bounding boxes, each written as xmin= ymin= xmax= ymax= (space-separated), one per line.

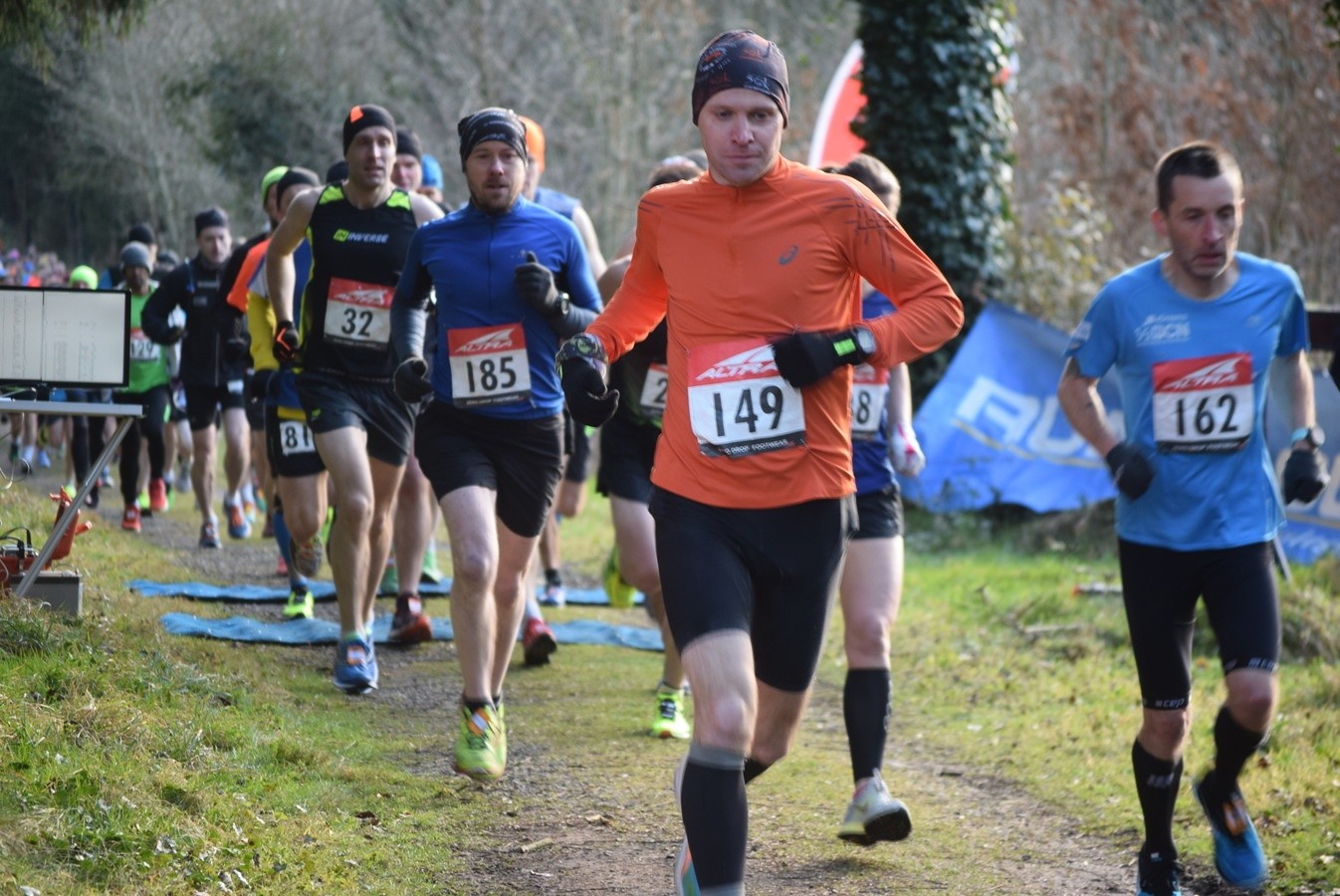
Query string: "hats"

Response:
xmin=688 ymin=23 xmax=790 ymax=129
xmin=519 ymin=116 xmax=546 ymax=168
xmin=69 ymin=107 xmax=443 ymax=289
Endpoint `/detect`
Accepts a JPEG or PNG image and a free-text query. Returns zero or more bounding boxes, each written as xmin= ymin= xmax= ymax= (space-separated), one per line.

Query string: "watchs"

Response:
xmin=1290 ymin=423 xmax=1328 ymax=455
xmin=850 ymin=319 xmax=879 ymax=360
xmin=551 ymin=287 xmax=572 ymax=325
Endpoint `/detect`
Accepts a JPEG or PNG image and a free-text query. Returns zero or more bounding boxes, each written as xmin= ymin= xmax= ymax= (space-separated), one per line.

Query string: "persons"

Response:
xmin=1059 ymin=141 xmax=1328 ymax=896
xmin=266 ymin=105 xmax=448 ymax=694
xmin=383 ymin=104 xmax=607 ymax=776
xmin=0 ymin=95 xmax=911 ymax=849
xmin=560 ymin=33 xmax=965 ymax=895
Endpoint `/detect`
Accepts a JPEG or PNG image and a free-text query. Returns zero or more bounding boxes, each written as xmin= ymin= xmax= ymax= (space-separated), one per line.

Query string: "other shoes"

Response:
xmin=252 ymin=482 xmax=268 ymax=512
xmin=243 ymin=500 xmax=257 ymax=524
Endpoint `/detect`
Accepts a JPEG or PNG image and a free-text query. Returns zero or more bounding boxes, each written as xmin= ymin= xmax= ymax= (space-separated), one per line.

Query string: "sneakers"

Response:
xmin=199 ymin=522 xmax=224 ymax=548
xmin=290 ymin=535 xmax=323 ymax=578
xmin=1135 ymin=840 xmax=1185 ymax=896
xmin=522 ymin=618 xmax=555 ymax=665
xmin=650 ymin=688 xmax=689 ymax=739
xmin=333 ymin=630 xmax=378 ymax=696
xmin=673 ymin=757 xmax=701 ymax=896
xmin=282 ymin=587 xmax=314 ymax=619
xmin=1194 ymin=771 xmax=1269 ymax=888
xmin=223 ymin=495 xmax=251 ymax=539
xmin=9 ymin=441 xmax=195 ymax=532
xmin=453 ymin=690 xmax=506 ymax=777
xmin=389 ymin=594 xmax=432 ymax=642
xmin=836 ymin=772 xmax=911 ymax=847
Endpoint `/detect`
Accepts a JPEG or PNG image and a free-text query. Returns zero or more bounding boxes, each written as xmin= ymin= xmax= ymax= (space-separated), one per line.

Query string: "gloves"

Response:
xmin=272 ymin=322 xmax=298 ymax=362
xmin=560 ymin=354 xmax=619 ymax=428
xmin=1283 ymin=448 xmax=1331 ymax=506
xmin=392 ymin=359 xmax=433 ymax=403
xmin=161 ymin=326 xmax=184 ymax=343
xmin=514 ymin=250 xmax=558 ymax=307
xmin=1106 ymin=445 xmax=1153 ymax=498
xmin=770 ymin=328 xmax=860 ymax=388
